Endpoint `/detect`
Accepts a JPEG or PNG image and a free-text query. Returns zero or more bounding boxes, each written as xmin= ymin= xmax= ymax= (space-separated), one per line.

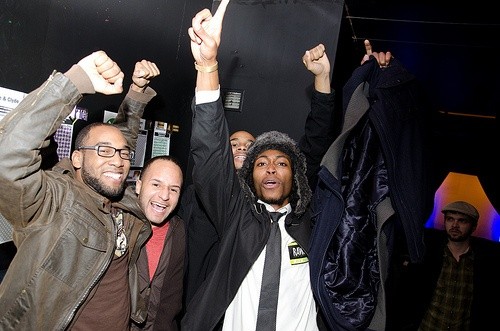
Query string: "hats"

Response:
xmin=441 ymin=201 xmax=479 ymax=219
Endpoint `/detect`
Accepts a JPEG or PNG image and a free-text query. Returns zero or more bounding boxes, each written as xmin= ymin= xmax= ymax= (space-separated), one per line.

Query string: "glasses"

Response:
xmin=78 ymin=146 xmax=135 ymax=160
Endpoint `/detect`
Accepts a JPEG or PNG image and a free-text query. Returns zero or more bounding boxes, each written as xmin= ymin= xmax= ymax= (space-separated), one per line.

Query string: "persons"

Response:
xmin=0 ymin=49 xmax=160 ymax=331
xmin=417 ymin=201 xmax=500 ymax=331
xmin=176 ymin=43 xmax=340 ymax=331
xmin=117 ymin=155 xmax=186 ymax=331
xmin=181 ymin=0 xmax=424 ymax=331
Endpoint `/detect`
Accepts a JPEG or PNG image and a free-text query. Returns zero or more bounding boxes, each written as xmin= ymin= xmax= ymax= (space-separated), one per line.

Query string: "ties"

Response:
xmin=256 ymin=211 xmax=287 ymax=331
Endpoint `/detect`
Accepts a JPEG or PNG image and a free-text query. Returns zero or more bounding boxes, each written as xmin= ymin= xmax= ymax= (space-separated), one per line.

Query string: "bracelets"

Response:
xmin=193 ymin=60 xmax=219 ymax=72
